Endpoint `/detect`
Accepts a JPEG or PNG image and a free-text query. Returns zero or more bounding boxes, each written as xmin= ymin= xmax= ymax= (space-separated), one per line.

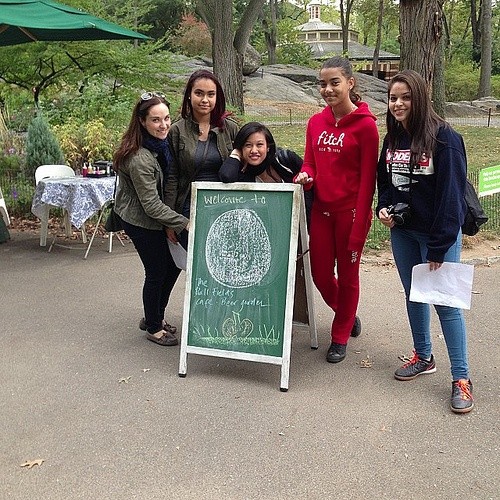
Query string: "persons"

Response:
xmin=113 ymin=97 xmax=190 ymax=346
xmin=164 ymin=70 xmax=240 ymax=244
xmin=377 ymin=70 xmax=474 ymax=413
xmin=293 ymin=57 xmax=380 ymax=363
xmin=219 ymin=122 xmax=313 ymax=233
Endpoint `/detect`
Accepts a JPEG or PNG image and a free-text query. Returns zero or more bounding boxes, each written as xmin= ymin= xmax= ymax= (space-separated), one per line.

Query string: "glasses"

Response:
xmin=140 ymin=91 xmax=166 ymax=104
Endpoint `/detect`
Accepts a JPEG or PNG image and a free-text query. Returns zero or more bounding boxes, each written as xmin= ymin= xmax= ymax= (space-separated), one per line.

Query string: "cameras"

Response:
xmin=386 ymin=203 xmax=414 ymax=227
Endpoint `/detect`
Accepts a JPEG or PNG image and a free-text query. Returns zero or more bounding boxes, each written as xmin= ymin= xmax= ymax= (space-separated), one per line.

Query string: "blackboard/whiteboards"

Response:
xmin=187 ymin=189 xmax=294 ymax=357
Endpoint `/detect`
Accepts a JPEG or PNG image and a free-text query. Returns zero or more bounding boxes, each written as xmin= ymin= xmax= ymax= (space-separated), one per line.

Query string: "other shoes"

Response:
xmin=351 ymin=316 xmax=361 ymax=338
xmin=139 ymin=317 xmax=177 ymax=334
xmin=145 ymin=328 xmax=178 ymax=346
xmin=327 ymin=342 xmax=346 ymax=362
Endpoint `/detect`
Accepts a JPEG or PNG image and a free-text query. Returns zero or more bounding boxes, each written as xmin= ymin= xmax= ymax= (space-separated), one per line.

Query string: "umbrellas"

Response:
xmin=0 ymin=0 xmax=155 ymax=47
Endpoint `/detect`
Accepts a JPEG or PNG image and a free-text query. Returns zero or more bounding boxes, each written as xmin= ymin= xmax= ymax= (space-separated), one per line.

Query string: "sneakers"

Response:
xmin=450 ymin=378 xmax=475 ymax=413
xmin=394 ymin=350 xmax=436 ymax=381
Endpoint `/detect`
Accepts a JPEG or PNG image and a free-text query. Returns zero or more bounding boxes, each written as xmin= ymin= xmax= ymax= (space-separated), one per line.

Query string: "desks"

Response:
xmin=31 ymin=176 xmax=124 ymax=252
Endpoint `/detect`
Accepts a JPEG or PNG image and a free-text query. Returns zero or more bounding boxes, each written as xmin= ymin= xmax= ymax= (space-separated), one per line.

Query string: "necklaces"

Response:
xmin=199 ymin=121 xmax=210 ymax=133
xmin=330 ymin=104 xmax=357 ymax=127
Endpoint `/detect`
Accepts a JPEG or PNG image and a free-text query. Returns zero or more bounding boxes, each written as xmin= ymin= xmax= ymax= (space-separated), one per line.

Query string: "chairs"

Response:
xmin=35 ymin=164 xmax=88 ymax=246
xmin=0 ymin=187 xmax=10 ymax=227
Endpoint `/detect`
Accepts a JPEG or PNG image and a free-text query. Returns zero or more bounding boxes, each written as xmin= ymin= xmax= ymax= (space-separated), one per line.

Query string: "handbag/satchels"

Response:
xmin=103 ymin=208 xmax=124 ymax=232
xmin=462 ymin=181 xmax=488 ymax=236
